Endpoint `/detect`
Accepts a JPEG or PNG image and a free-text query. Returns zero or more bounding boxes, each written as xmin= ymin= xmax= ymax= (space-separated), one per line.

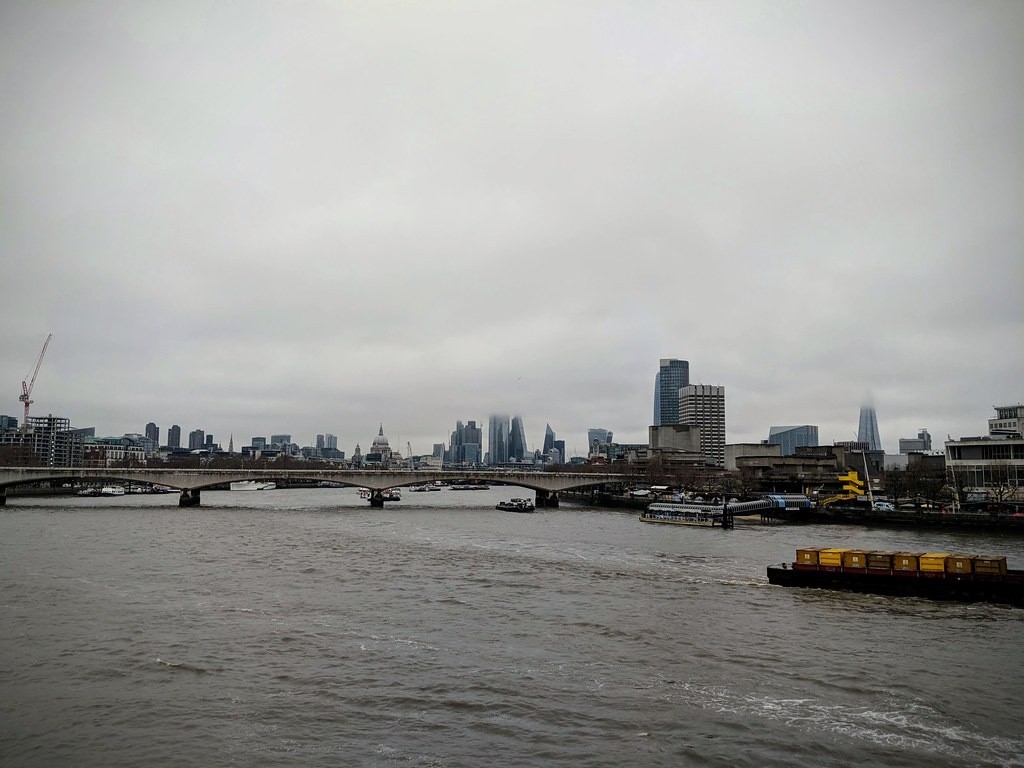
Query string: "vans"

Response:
xmin=874 ymin=502 xmax=895 ymax=511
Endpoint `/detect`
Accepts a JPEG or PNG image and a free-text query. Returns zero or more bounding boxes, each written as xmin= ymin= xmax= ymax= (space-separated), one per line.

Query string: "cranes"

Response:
xmin=18 ymin=333 xmax=53 ymax=423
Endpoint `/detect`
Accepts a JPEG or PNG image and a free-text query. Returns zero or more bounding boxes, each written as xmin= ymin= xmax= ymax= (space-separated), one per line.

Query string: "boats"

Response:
xmin=357 ymin=488 xmax=401 ymax=501
xmin=452 ymin=484 xmax=490 ymax=491
xmin=409 ymin=484 xmax=441 ymax=492
xmin=640 ymin=503 xmax=723 ymax=528
xmin=79 ymin=486 xmax=125 ymax=497
xmin=495 ymin=497 xmax=535 ymax=512
